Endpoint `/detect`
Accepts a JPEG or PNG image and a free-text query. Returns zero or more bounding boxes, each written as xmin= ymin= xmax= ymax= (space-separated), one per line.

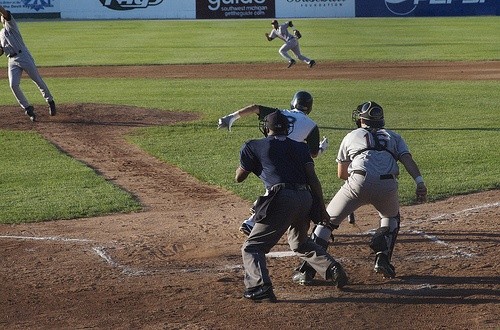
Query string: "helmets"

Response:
xmin=259 ymin=111 xmax=297 ymax=137
xmin=291 ymin=90 xmax=312 ymax=115
xmin=352 ymin=101 xmax=385 ymax=127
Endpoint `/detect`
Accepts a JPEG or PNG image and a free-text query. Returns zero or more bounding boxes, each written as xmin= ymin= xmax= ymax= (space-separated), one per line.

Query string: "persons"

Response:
xmin=217 ymin=91 xmax=348 ymax=302
xmin=292 ymin=101 xmax=428 ymax=286
xmin=0 ymin=4 xmax=56 ymax=121
xmin=265 ymin=20 xmax=315 ymax=67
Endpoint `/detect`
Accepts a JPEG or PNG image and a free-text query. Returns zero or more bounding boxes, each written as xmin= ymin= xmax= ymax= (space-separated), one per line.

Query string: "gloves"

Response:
xmin=316 ymin=136 xmax=330 ymax=159
xmin=217 ymin=111 xmax=240 ymax=133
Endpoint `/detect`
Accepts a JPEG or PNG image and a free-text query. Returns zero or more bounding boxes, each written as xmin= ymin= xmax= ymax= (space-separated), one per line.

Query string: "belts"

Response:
xmin=269 ymin=183 xmax=309 ymax=192
xmin=9 ymin=50 xmax=22 ymax=57
xmin=353 ymin=170 xmax=394 ymax=179
xmin=288 ymin=37 xmax=294 ymax=40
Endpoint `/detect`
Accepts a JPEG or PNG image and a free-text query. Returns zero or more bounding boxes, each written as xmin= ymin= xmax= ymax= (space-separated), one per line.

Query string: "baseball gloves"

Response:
xmin=294 ymin=30 xmax=302 ymax=39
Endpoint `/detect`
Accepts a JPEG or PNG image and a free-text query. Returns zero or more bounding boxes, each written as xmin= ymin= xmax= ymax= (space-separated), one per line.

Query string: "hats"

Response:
xmin=270 ymin=20 xmax=278 ymax=24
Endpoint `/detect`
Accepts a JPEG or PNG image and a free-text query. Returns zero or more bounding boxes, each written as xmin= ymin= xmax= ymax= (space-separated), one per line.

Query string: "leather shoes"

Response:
xmin=332 ymin=264 xmax=348 ymax=288
xmin=244 ymin=286 xmax=276 ymax=303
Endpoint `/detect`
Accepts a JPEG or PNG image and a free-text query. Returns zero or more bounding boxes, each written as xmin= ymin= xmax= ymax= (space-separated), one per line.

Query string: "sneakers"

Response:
xmin=374 ymin=255 xmax=396 ymax=279
xmin=309 ymin=59 xmax=315 ymax=69
xmin=238 ymin=220 xmax=254 ymax=237
xmin=288 ymin=59 xmax=296 ymax=68
xmin=48 ymin=100 xmax=56 ymax=116
xmin=293 ymin=268 xmax=313 ymax=285
xmin=24 ymin=106 xmax=36 ymax=122
